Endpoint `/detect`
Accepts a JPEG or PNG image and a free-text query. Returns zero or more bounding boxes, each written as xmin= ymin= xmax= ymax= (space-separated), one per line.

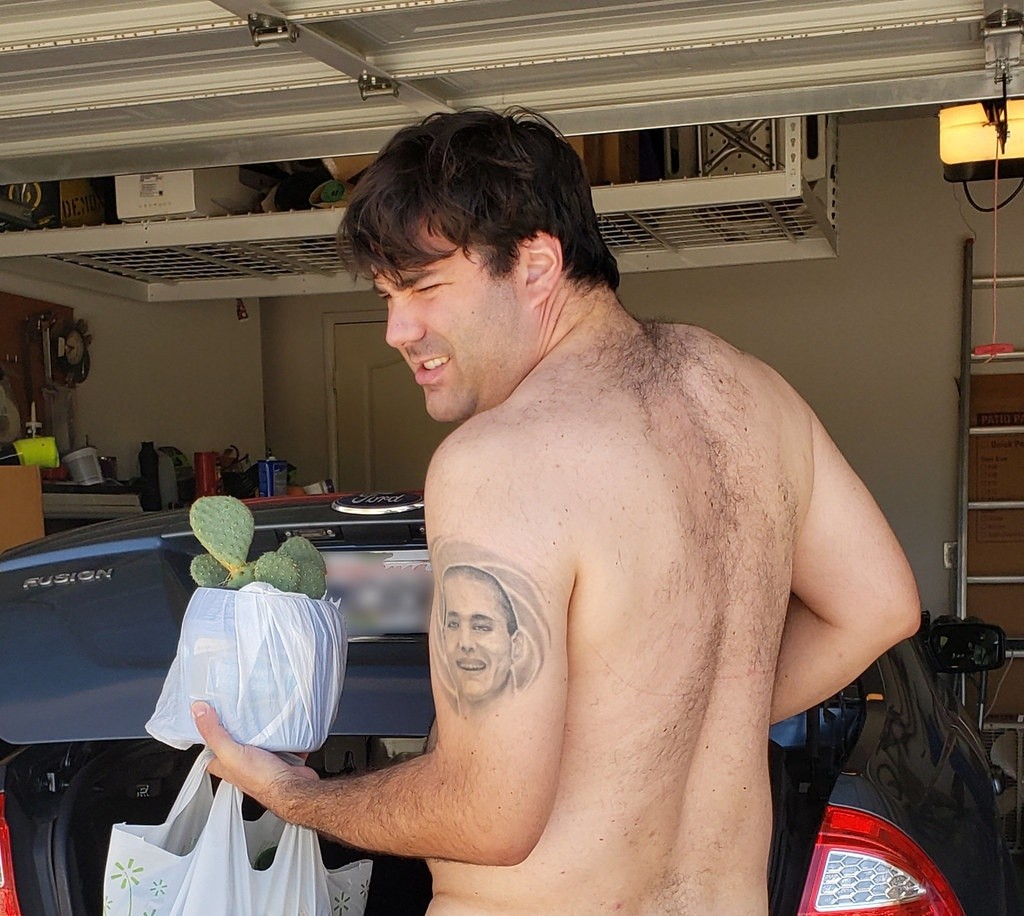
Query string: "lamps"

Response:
xmin=933 ymin=97 xmax=1024 ymax=212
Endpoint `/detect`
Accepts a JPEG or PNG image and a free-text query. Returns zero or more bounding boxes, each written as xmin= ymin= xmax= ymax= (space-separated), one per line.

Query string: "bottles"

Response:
xmin=257 ymin=456 xmax=288 ymax=498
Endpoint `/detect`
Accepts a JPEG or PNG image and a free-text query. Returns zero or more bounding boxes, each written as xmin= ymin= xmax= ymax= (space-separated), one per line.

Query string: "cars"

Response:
xmin=1 ymin=492 xmax=1014 ymax=916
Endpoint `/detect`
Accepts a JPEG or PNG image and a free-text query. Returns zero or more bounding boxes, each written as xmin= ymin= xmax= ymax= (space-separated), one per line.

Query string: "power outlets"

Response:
xmin=944 ymin=542 xmax=958 ymax=569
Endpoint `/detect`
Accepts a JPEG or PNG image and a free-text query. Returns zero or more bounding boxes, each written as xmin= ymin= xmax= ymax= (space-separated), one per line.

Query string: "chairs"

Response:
xmin=51 ymin=742 xmax=273 ymax=916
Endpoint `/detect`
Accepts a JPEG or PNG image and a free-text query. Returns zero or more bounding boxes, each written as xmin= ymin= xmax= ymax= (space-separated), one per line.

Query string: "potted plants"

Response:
xmin=144 ymin=493 xmax=347 ymax=752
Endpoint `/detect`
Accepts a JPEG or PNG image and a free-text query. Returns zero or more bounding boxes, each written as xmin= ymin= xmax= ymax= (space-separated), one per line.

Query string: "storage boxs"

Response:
xmin=114 ymin=167 xmax=263 ymax=223
xmin=950 ymin=372 xmax=1024 ymax=717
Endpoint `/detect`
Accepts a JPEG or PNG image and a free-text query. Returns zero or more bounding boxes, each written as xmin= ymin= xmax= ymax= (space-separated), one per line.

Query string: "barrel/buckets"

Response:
xmin=62 ymin=446 xmax=104 ymax=486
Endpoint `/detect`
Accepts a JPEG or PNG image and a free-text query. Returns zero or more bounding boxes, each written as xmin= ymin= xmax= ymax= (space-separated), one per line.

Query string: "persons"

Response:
xmin=191 ymin=107 xmax=923 ymax=916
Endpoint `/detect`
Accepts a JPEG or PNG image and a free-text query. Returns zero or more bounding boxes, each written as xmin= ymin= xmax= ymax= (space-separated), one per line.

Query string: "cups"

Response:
xmin=303 ymin=479 xmax=334 ymax=494
